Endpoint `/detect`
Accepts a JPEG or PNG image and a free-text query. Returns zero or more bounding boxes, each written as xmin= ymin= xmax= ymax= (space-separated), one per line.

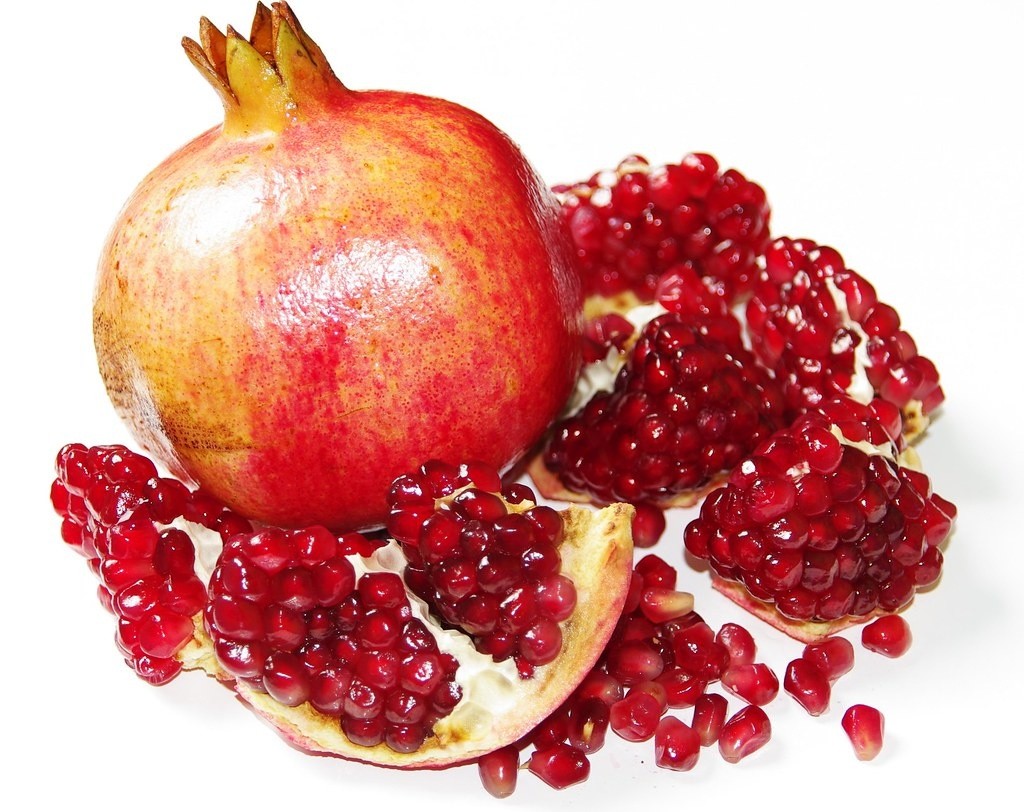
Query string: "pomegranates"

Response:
xmin=49 ymin=0 xmax=957 ymax=800
